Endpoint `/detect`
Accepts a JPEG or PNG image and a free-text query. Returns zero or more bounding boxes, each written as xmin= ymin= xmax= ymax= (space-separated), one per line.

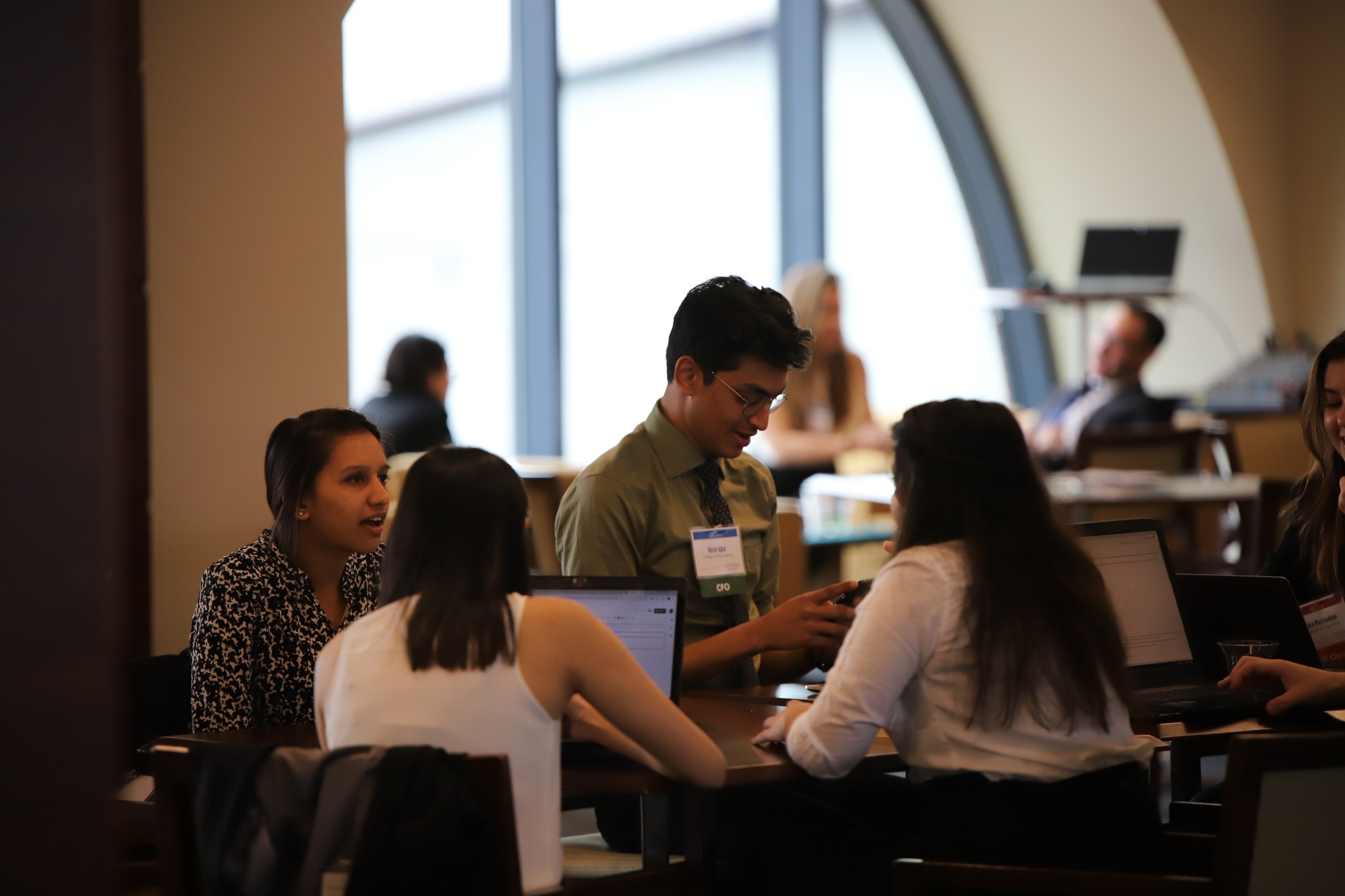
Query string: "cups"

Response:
xmin=1218 ymin=641 xmax=1280 ymax=689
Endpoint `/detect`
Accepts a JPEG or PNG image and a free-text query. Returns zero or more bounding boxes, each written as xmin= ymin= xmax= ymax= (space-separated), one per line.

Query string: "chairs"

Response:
xmin=887 ymin=730 xmax=1344 ymax=895
xmin=147 ymin=744 xmax=712 ymax=896
xmin=1074 ymin=427 xmax=1205 ymax=570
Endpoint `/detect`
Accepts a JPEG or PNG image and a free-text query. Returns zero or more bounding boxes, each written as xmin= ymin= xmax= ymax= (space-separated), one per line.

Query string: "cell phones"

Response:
xmin=832 ymin=578 xmax=876 ymax=610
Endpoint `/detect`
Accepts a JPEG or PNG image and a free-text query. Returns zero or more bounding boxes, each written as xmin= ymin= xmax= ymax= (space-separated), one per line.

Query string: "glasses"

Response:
xmin=711 ymin=371 xmax=789 ymax=416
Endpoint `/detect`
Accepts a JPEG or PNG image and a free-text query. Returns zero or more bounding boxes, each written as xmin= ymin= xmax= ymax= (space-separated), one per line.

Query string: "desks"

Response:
xmin=412 ymin=449 xmax=845 ymax=575
xmin=601 ymin=642 xmax=1344 ymax=896
xmin=776 ymin=469 xmax=1263 ymax=575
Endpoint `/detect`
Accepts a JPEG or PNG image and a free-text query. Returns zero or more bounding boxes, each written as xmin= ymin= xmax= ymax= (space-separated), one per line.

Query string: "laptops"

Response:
xmin=1174 ymin=571 xmax=1327 ymax=681
xmin=1064 ymin=517 xmax=1281 ymax=732
xmin=525 ymin=572 xmax=686 ymax=728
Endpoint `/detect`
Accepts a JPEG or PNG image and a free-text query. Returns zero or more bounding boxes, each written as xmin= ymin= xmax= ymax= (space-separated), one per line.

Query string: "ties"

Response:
xmin=694 ymin=463 xmax=761 ymax=686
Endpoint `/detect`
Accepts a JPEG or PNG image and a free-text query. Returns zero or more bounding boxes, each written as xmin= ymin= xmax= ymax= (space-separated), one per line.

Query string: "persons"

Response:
xmin=1217 ymin=654 xmax=1345 ymax=715
xmin=751 ymin=397 xmax=1165 ymax=896
xmin=1023 ymin=297 xmax=1166 ymax=469
xmin=1260 ymin=331 xmax=1345 ymax=671
xmin=553 ymin=277 xmax=860 ymax=855
xmin=359 ymin=337 xmax=455 ymax=455
xmin=189 ymin=408 xmax=390 ymax=732
xmin=742 ymin=260 xmax=894 ymax=498
xmin=313 ymin=448 xmax=727 ymax=896
